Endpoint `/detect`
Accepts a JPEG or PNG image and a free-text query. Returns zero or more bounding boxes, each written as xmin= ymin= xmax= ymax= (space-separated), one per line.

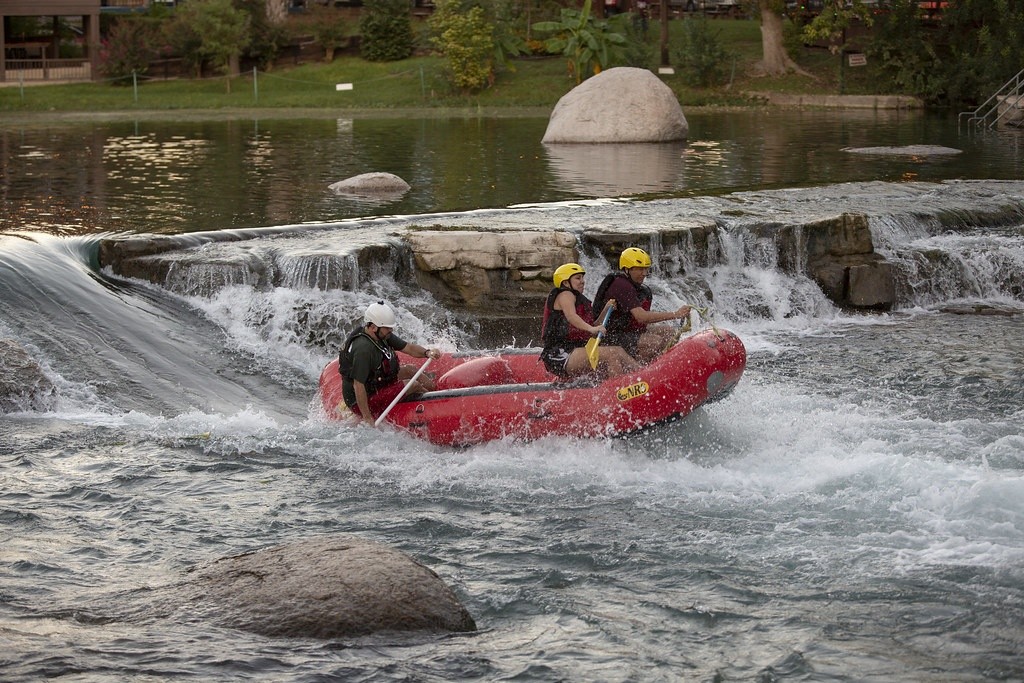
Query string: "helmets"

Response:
xmin=620 ymin=248 xmax=651 ymax=269
xmin=364 ymin=303 xmax=396 ymax=328
xmin=554 ymin=264 xmax=585 ymax=288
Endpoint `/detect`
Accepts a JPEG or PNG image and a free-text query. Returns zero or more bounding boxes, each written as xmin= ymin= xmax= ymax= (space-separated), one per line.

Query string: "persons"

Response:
xmin=538 ymin=263 xmax=644 ymax=380
xmin=592 ymin=248 xmax=692 ymax=365
xmin=338 ymin=300 xmax=442 ymax=429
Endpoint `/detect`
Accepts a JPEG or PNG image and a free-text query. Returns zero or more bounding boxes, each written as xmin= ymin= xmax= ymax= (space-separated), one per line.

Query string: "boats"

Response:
xmin=318 ymin=329 xmax=747 ymax=448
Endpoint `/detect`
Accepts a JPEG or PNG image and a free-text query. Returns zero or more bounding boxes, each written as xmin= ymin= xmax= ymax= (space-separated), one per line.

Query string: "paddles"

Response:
xmin=586 ymin=304 xmax=611 ymax=370
xmin=374 ymin=356 xmax=431 ymax=427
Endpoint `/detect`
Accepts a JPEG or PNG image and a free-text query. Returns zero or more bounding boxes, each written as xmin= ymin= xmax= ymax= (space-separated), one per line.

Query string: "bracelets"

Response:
xmin=424 ymin=349 xmax=430 ymax=358
